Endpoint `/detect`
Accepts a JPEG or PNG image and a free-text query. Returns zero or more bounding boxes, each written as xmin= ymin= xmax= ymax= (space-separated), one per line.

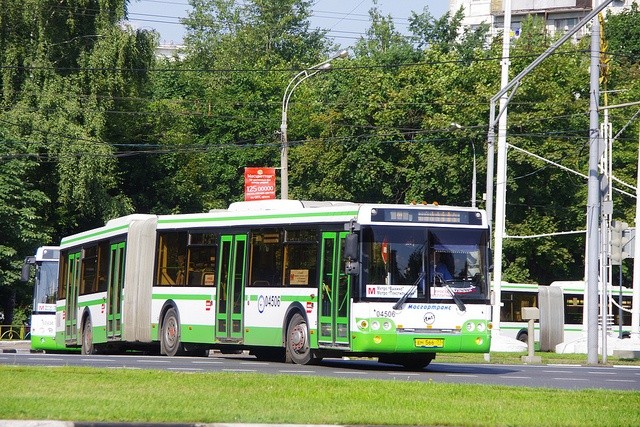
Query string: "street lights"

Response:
xmin=450 ymin=122 xmax=477 ymax=207
xmin=281 ymin=50 xmax=348 ymax=199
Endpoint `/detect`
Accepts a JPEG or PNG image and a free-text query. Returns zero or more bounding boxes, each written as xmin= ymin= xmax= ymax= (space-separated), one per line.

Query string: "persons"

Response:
xmin=419 ymin=251 xmax=453 ymax=289
xmin=175 ymin=254 xmax=185 ymax=286
xmin=278 ymin=270 xmax=291 ymax=287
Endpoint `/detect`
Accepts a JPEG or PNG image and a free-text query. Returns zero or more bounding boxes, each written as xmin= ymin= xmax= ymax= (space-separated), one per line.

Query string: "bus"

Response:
xmin=22 ymin=245 xmax=82 ymax=354
xmin=490 ymin=280 xmax=640 ymax=352
xmin=55 ymin=199 xmax=493 ymax=368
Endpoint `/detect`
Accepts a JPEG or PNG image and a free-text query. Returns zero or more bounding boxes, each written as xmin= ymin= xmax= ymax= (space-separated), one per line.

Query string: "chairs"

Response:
xmin=253 ymin=280 xmax=270 ymax=287
xmin=187 ymin=270 xmax=201 ymax=285
xmin=515 ymin=311 xmax=521 ymax=321
